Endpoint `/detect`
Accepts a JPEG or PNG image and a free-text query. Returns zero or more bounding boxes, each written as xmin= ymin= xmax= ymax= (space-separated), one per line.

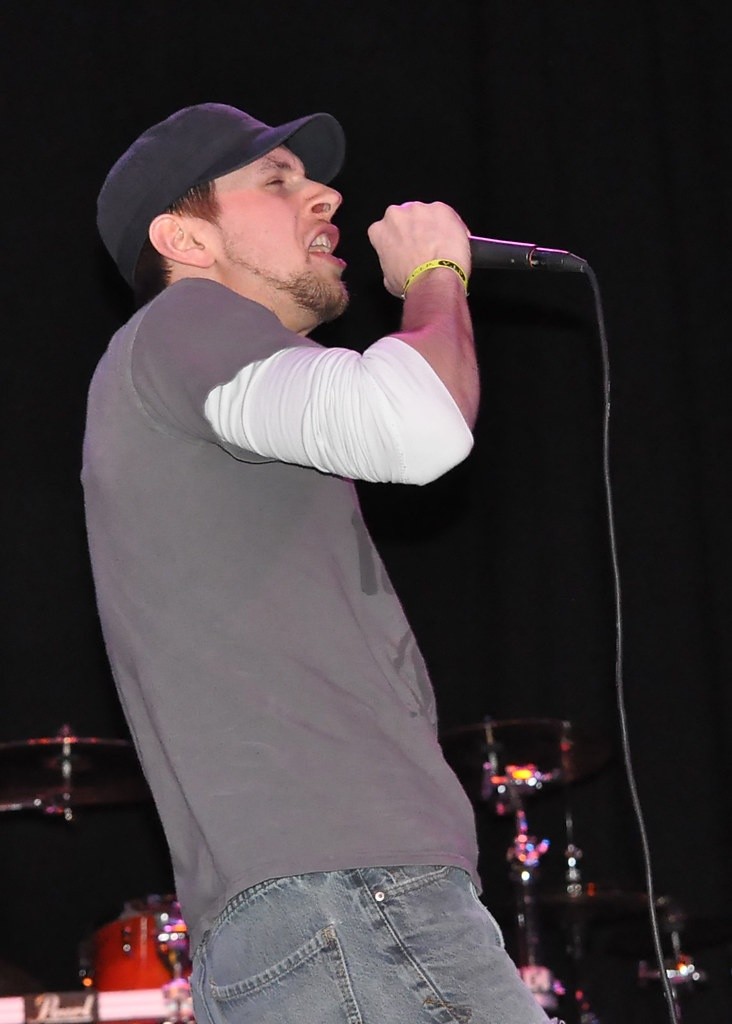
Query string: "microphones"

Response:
xmin=468 ymin=235 xmax=588 ymax=272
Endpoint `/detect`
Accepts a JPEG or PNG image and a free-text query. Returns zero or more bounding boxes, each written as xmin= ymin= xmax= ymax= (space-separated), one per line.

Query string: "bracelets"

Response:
xmin=402 ymin=258 xmax=467 ymax=299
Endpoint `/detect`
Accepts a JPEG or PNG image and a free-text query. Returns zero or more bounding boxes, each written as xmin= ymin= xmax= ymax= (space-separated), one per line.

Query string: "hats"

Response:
xmin=96 ymin=102 xmax=344 ymax=293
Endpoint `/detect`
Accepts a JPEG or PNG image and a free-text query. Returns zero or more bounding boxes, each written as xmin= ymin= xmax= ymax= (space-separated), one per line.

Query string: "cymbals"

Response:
xmin=506 ymin=885 xmax=662 ymax=929
xmin=450 ymin=714 xmax=556 ymax=735
xmin=5 ymin=730 xmax=132 ymax=753
xmin=2 ymin=783 xmax=148 ymax=812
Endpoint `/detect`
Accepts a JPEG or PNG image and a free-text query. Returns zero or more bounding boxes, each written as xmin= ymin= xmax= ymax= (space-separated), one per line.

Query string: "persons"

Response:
xmin=83 ymin=103 xmax=549 ymax=1024
